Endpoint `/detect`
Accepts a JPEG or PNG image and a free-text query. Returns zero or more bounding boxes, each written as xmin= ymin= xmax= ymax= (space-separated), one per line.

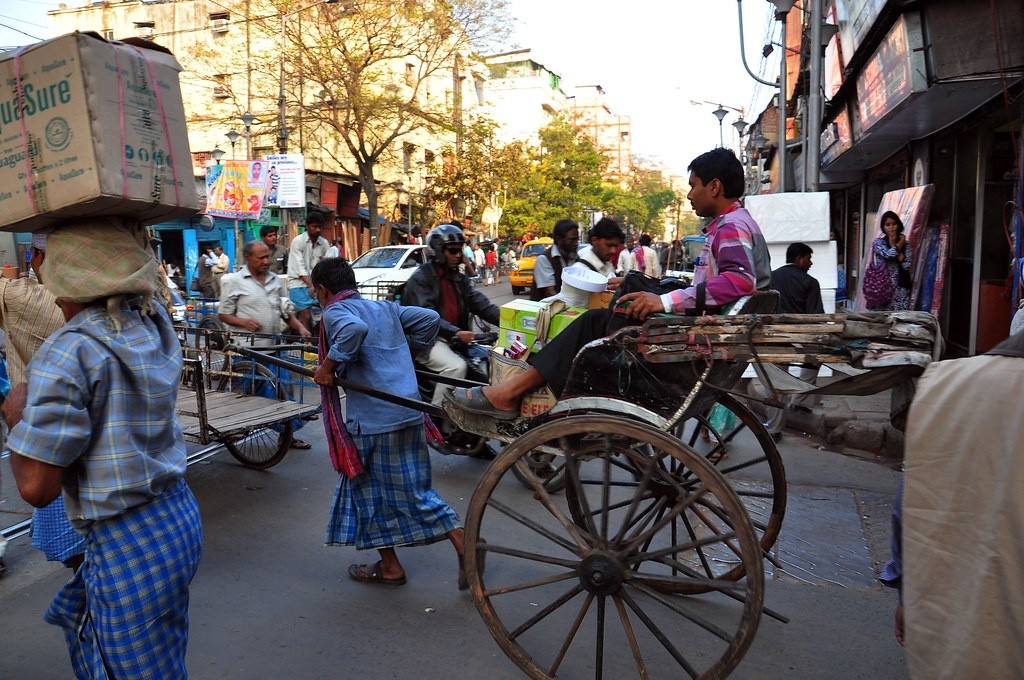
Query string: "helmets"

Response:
xmin=427 ymin=225 xmax=466 ymax=253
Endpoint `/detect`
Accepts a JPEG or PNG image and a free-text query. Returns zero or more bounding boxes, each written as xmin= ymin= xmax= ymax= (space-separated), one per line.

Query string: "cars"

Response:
xmin=650 ymin=241 xmax=676 ymax=264
xmin=576 ymin=243 xmax=591 ymax=253
xmin=350 ymin=245 xmax=428 ymax=302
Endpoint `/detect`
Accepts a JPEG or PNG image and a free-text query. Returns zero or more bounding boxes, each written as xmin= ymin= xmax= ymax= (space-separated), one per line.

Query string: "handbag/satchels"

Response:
xmin=608 ymin=270 xmax=694 ymax=314
xmin=191 ymin=277 xmax=201 ymax=291
xmin=862 ymin=238 xmax=894 ymax=306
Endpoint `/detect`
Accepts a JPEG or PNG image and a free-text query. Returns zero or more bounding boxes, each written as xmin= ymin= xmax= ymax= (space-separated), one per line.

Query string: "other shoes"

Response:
xmin=470 ymin=444 xmax=497 ymax=460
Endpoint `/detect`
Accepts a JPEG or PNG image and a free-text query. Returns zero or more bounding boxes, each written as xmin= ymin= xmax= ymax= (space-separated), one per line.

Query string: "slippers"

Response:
xmin=278 ymin=439 xmax=311 ymax=449
xmin=709 ymin=457 xmax=719 ymax=461
xmin=701 ymin=430 xmax=710 ymax=442
xmin=443 ymin=386 xmax=518 ymax=419
xmin=458 ymin=538 xmax=486 ymax=590
xmin=349 ymin=560 xmax=407 ymax=586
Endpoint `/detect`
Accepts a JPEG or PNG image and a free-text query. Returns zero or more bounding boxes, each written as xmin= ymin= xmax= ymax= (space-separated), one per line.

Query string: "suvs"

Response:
xmin=510 ymin=236 xmax=556 ymax=296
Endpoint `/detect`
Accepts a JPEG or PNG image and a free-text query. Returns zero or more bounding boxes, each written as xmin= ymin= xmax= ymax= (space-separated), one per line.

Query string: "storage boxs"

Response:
xmin=741 ymin=191 xmax=839 ymax=378
xmin=499 ymin=299 xmax=588 ymax=353
xmin=0 ymin=29 xmax=201 ymax=232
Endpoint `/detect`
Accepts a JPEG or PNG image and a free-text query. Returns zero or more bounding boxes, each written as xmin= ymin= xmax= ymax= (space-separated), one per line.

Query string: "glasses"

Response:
xmin=446 ymin=247 xmax=462 ymax=255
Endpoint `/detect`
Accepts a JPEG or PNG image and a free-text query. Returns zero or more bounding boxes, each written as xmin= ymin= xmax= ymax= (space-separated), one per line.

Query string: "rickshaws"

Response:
xmin=222 ymin=290 xmax=790 ymax=679
xmin=164 ymin=297 xmax=326 ymax=473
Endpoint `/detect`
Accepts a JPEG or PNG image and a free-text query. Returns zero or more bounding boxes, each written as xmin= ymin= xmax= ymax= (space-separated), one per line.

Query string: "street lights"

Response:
xmin=493 ymin=190 xmax=501 ymax=239
xmin=393 ymin=179 xmax=404 ymax=223
xmin=767 ymin=0 xmax=800 ymax=192
xmin=404 ymin=169 xmax=415 ymax=245
xmin=731 ymin=114 xmax=750 ymax=168
xmin=591 ymin=208 xmax=595 ymax=229
xmin=711 ymin=103 xmax=729 ymax=149
xmin=224 ymin=127 xmax=240 ymax=160
xmin=240 ymin=110 xmax=255 ymax=161
xmin=750 ymin=133 xmax=769 ymax=196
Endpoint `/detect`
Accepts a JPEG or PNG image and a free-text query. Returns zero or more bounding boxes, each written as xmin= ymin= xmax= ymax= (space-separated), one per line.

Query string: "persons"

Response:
xmin=861 ymin=210 xmax=912 ymax=312
xmin=216 ymin=240 xmax=311 ymax=450
xmin=0 ymin=230 xmax=86 ymax=577
xmin=198 ymin=246 xmax=230 ymax=302
xmin=616 ymin=234 xmax=659 ymax=279
xmin=165 ymin=260 xmax=183 ymax=277
xmin=268 ymin=166 xmax=280 ymax=204
xmin=529 ymin=219 xmax=578 ymax=299
xmin=1 ymin=217 xmax=202 ymax=679
xmin=574 ymin=218 xmax=625 ymax=289
xmin=440 ymin=149 xmax=772 ymax=419
xmin=401 ymin=225 xmax=500 ymax=459
xmin=259 ymin=211 xmax=346 ymax=347
xmin=658 ymin=240 xmax=683 ymax=274
xmin=877 ymin=330 xmax=1024 ymax=680
xmin=767 ymin=241 xmax=827 ymax=443
xmin=451 ymin=220 xmax=538 ymax=286
xmin=387 ymin=234 xmax=423 ymax=247
xmin=249 ymin=162 xmax=262 ymax=183
xmin=311 ymin=259 xmax=487 ymax=590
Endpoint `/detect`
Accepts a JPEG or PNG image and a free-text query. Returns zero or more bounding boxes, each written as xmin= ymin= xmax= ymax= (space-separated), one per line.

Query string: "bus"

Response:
xmin=683 ymin=234 xmax=708 ymax=272
